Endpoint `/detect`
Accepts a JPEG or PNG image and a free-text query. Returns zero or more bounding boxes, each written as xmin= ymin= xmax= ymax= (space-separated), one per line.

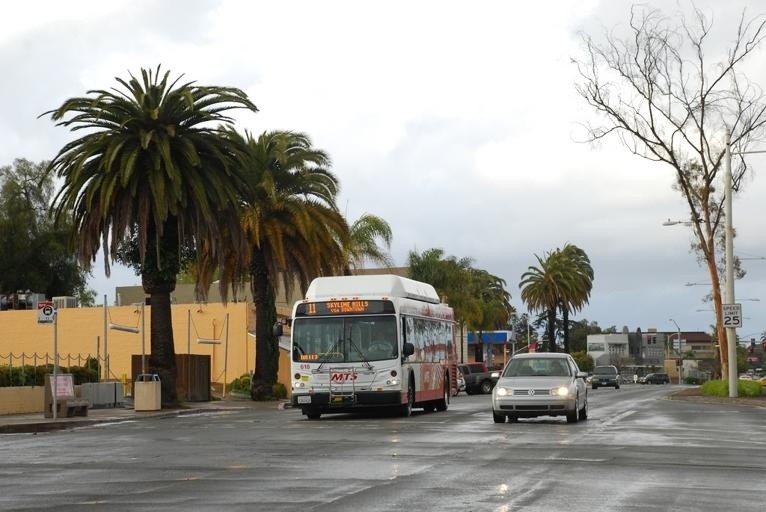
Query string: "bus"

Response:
xmin=271 ymin=273 xmax=458 ymax=422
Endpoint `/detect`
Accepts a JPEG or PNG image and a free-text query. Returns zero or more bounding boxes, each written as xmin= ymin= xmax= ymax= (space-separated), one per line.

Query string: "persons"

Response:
xmin=633 ymin=372 xmax=638 ymax=383
xmin=368 ymin=330 xmax=394 ymax=359
xmin=548 ymin=360 xmax=567 ymax=376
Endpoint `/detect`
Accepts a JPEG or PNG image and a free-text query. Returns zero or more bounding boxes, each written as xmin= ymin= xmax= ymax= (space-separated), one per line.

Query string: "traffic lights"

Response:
xmin=762 ymin=342 xmax=766 ymax=351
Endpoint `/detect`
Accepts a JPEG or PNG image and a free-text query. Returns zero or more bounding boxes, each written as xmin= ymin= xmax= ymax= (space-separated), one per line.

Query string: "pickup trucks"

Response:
xmin=457 ymin=362 xmax=504 ymax=394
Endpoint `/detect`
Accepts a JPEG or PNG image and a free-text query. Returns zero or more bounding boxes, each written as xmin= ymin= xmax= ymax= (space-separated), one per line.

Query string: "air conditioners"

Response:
xmin=51 ymin=295 xmax=75 ymax=308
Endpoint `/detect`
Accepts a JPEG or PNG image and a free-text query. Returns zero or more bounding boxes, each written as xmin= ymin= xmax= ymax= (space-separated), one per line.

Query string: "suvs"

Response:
xmin=591 ymin=365 xmax=621 ymax=389
xmin=637 ymin=373 xmax=669 ymax=385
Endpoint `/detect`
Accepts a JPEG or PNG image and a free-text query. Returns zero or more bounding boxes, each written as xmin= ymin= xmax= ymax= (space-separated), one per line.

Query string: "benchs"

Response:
xmin=42 ymin=372 xmax=89 ymax=419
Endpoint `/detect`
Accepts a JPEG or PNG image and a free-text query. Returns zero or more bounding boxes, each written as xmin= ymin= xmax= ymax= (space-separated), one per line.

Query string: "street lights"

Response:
xmin=522 ymin=313 xmax=530 ymax=348
xmin=683 ymin=282 xmax=724 ymax=287
xmin=669 ymin=319 xmax=682 ymax=385
xmin=666 ymin=332 xmax=681 ymax=374
xmin=663 ymin=218 xmax=724 ymax=229
xmin=696 ymin=308 xmax=717 ymax=313
xmin=743 ymin=316 xmax=752 ymax=321
xmin=734 ymin=298 xmax=760 ymax=302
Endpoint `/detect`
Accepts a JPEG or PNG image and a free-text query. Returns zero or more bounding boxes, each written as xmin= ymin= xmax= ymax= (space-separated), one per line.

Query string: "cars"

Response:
xmin=489 ymin=352 xmax=590 ymax=423
xmin=453 ymin=366 xmax=466 ymax=396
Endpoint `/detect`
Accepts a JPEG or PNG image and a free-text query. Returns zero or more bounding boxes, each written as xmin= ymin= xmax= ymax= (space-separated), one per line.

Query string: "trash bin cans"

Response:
xmin=134 ymin=374 xmax=162 ymax=412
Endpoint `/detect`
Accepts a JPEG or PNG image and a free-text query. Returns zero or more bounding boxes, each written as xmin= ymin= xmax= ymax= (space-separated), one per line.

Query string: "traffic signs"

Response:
xmin=721 ymin=304 xmax=742 ymax=328
